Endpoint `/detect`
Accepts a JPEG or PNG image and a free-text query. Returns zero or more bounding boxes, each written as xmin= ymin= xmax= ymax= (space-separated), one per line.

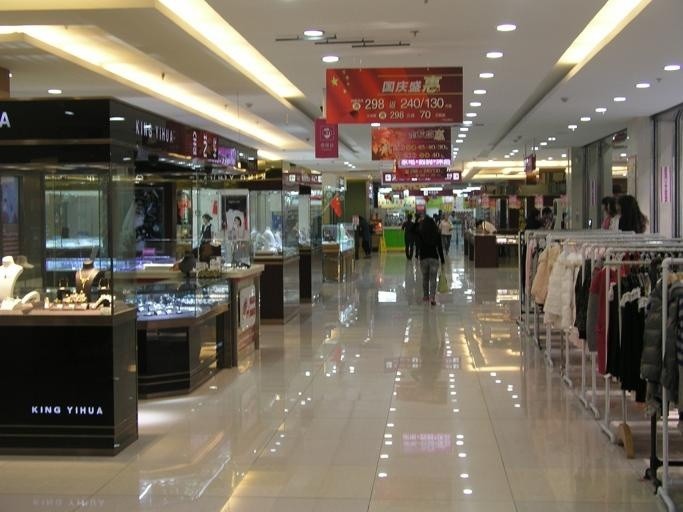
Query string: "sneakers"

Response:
xmin=422 ymin=295 xmax=436 ymax=305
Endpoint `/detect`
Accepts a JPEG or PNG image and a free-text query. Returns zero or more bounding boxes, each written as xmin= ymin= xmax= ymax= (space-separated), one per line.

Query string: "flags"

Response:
xmin=330 ymin=195 xmax=342 ymax=219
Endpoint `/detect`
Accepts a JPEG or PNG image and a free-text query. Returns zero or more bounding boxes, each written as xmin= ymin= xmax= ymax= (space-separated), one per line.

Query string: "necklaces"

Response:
xmin=76 ymin=265 xmax=94 ymax=290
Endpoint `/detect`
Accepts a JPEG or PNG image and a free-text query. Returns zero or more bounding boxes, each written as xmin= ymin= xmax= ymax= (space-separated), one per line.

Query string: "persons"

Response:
xmin=599 ymin=197 xmax=613 ymax=227
xmin=351 ymin=212 xmax=373 ymax=259
xmin=607 ymin=195 xmax=624 ymax=231
xmin=418 ymin=218 xmax=445 ymax=305
xmin=541 ymin=207 xmax=553 ymax=230
xmin=197 ymin=213 xmax=214 ymax=247
xmin=559 ymin=211 xmax=567 ymax=229
xmin=524 ymin=207 xmax=540 ymax=227
xmin=226 ymin=215 xmax=244 ymax=238
xmin=618 ymin=194 xmax=647 ymax=234
xmin=401 ymin=210 xmax=457 ymax=261
xmin=475 ymin=219 xmax=496 ymax=234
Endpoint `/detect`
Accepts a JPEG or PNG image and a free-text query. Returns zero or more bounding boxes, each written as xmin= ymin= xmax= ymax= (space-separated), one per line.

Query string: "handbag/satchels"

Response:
xmin=438 ymin=273 xmax=448 ymax=293
